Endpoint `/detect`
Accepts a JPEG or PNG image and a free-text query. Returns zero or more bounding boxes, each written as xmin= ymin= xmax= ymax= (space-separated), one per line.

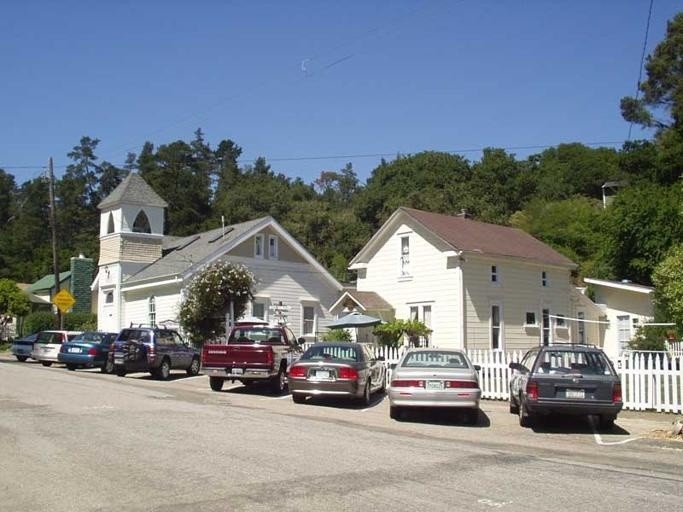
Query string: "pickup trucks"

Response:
xmin=201 ymin=321 xmax=305 ymax=390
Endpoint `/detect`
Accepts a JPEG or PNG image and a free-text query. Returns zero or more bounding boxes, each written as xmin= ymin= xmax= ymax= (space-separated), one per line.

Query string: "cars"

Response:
xmin=509 ymin=343 xmax=623 ymax=427
xmin=390 ymin=345 xmax=481 ymax=419
xmin=10 ymin=323 xmax=200 ymax=380
xmin=286 ymin=342 xmax=386 ymax=405
